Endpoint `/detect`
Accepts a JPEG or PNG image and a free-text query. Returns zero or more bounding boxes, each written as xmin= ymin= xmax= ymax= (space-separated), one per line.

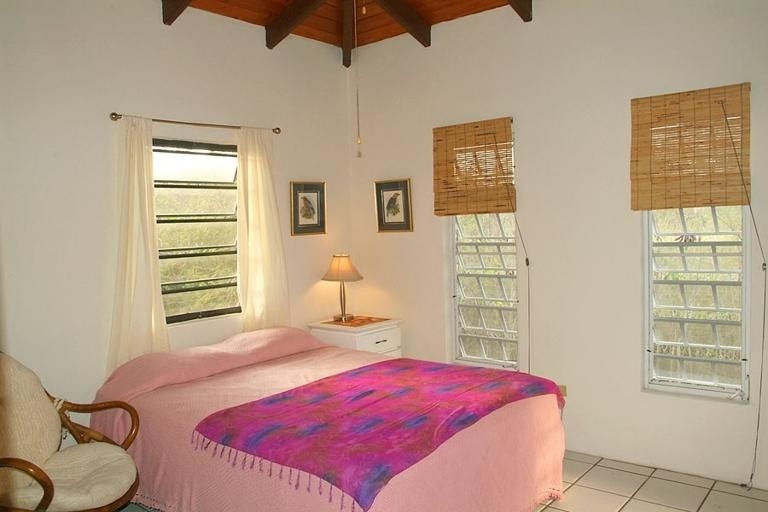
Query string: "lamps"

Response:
xmin=321 ymin=254 xmax=364 ymax=321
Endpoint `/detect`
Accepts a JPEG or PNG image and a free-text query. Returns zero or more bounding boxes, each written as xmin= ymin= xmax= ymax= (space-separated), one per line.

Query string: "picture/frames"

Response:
xmin=290 ymin=181 xmax=326 ymax=236
xmin=374 ymin=178 xmax=413 ymax=233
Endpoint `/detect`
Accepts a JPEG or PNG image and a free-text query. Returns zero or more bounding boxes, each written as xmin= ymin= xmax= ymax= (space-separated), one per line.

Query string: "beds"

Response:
xmin=89 ymin=327 xmax=565 ymax=512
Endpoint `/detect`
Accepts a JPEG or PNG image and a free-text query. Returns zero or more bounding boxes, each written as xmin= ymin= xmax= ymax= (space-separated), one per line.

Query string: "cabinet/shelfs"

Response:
xmin=308 ymin=315 xmax=402 ymax=358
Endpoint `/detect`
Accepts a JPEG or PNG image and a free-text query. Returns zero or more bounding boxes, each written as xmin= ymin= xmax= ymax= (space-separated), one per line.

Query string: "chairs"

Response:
xmin=0 ymin=351 xmax=139 ymax=512
